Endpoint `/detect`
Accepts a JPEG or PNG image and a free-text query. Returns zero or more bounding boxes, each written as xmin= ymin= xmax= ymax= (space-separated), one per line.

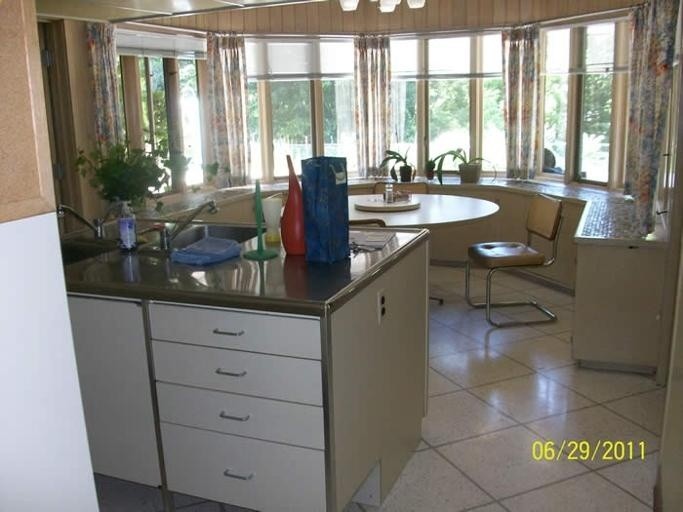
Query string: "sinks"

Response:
xmin=140 ymin=224 xmax=267 ymax=257
xmin=61 ymin=240 xmax=119 ymax=266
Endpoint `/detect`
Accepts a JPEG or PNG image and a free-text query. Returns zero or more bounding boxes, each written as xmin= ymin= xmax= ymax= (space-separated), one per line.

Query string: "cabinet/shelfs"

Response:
xmin=197 ymin=194 xmax=260 ymax=225
xmin=569 ymin=202 xmax=675 ymax=376
xmin=147 ymin=221 xmax=432 ymax=510
xmin=499 ymin=188 xmax=585 ymax=293
xmin=65 ymin=293 xmax=166 ymax=485
xmin=426 ymin=182 xmax=498 ymax=272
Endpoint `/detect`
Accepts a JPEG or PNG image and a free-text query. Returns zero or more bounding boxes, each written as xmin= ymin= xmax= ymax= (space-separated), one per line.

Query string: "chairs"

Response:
xmin=463 ymin=190 xmax=567 ymax=328
xmin=371 ymin=181 xmax=429 ymax=196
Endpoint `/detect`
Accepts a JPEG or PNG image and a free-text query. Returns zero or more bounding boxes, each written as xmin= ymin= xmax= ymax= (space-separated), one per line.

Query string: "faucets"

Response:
xmin=159 ymin=199 xmax=219 ymax=250
xmin=57 ymin=203 xmax=104 ymax=238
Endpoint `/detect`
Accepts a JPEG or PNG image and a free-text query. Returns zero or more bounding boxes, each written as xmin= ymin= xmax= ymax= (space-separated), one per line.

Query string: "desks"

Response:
xmin=343 ymin=191 xmax=500 ymax=307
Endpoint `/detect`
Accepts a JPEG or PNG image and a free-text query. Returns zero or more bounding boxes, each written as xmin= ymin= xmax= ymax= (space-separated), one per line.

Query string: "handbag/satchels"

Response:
xmin=301 ymin=156 xmax=349 ymax=264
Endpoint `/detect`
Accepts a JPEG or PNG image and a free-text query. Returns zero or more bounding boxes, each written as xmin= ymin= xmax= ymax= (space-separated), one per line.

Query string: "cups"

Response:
xmin=261 ymin=198 xmax=283 ymax=242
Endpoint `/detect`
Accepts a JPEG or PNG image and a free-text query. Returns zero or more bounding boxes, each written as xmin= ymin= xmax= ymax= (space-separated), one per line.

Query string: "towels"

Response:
xmin=169 ymin=232 xmax=243 ymax=269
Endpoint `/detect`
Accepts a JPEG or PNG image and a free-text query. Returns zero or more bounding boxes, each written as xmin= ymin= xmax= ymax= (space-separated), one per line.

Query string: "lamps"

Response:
xmin=335 ymin=0 xmax=426 ymax=17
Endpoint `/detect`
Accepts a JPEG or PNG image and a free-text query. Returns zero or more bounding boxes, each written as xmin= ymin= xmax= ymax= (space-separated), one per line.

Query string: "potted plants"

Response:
xmin=423 ymin=159 xmax=434 ymax=181
xmin=430 ymin=147 xmax=496 ymax=186
xmin=381 ymin=147 xmax=418 ymax=183
xmin=74 ymin=129 xmax=186 ymax=254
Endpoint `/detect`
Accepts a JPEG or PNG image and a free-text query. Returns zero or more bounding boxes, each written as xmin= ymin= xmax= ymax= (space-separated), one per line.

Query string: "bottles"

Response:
xmin=280 ymin=154 xmax=303 ymax=256
xmin=384 ymin=184 xmax=394 ymax=203
xmin=116 ymin=200 xmax=136 ymax=252
